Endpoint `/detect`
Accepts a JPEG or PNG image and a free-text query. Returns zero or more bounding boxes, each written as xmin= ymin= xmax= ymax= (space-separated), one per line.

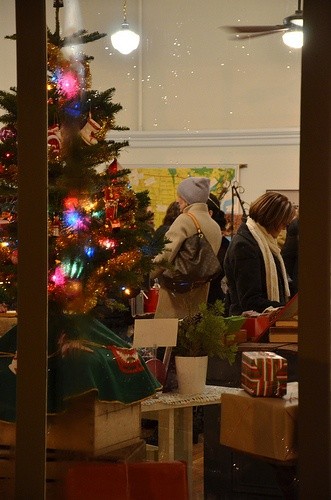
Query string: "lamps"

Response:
xmin=111 ymin=0 xmax=140 ymax=55
xmin=283 ymin=0 xmax=305 ymax=50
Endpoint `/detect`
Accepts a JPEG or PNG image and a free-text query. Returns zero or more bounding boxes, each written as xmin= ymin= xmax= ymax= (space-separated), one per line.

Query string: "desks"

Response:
xmin=140 ymin=385 xmax=249 ymax=500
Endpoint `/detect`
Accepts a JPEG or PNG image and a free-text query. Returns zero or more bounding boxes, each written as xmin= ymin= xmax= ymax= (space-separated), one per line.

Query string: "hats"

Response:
xmin=206 ymin=192 xmax=220 ymax=212
xmin=177 ymin=177 xmax=210 ymax=204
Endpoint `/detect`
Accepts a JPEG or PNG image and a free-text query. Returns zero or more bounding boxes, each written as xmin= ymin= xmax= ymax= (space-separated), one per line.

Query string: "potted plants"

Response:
xmin=174 ymin=300 xmax=238 ymax=394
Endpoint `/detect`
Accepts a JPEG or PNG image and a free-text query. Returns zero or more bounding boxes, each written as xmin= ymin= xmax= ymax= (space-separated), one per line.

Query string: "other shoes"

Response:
xmin=145 ymin=432 xmax=198 ymax=446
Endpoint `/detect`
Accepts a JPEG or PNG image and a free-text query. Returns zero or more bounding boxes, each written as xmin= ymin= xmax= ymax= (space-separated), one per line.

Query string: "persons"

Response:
xmin=279 ymin=204 xmax=297 ymax=299
xmin=152 ymin=198 xmax=183 ymax=250
xmin=201 ymin=191 xmax=230 ymax=309
xmin=224 ymin=191 xmax=295 ymax=324
xmin=146 ymin=175 xmax=222 ymax=366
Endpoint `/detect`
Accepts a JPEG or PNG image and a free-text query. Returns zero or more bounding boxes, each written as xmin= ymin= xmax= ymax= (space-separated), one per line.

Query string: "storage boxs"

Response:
xmin=0 ymin=313 xmax=298 ymax=500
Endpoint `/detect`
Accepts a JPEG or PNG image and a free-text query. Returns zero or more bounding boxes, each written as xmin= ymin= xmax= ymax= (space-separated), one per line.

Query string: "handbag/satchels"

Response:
xmin=162 ymin=212 xmax=223 ymax=297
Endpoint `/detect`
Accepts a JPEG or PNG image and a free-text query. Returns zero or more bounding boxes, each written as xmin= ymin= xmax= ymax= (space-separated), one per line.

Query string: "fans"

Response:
xmin=221 ymin=0 xmax=304 ymax=41
